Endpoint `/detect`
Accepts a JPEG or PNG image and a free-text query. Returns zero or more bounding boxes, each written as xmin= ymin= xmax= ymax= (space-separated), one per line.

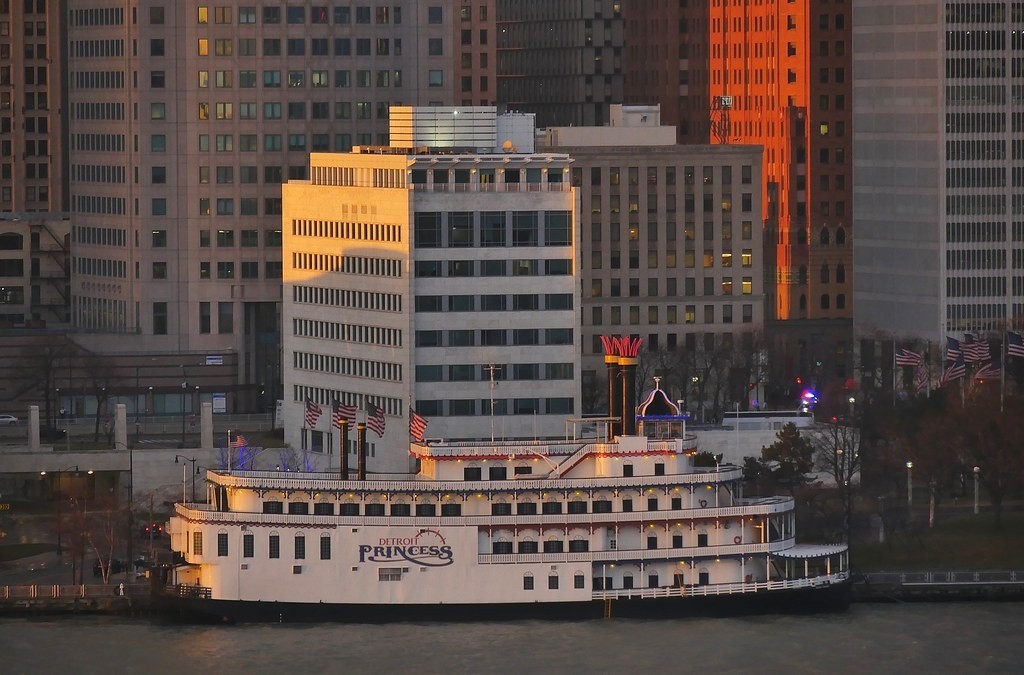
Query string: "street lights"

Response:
xmin=906 ymin=460 xmax=913 ymax=506
xmin=174 ymin=454 xmax=197 ymax=502
xmin=973 ymin=465 xmax=981 ymax=515
xmin=40 ymin=465 xmax=79 ymax=558
xmin=107 ymin=441 xmax=134 ymax=572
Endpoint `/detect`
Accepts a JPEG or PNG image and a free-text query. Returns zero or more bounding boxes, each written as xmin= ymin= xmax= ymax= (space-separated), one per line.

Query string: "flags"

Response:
xmin=300 ymin=393 xmax=430 ymax=441
xmin=230 ymin=433 xmax=249 ymax=448
xmin=892 ymin=330 xmax=1024 ymax=393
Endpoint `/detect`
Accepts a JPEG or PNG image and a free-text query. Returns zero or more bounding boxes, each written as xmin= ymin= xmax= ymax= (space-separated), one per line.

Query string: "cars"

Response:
xmin=91 ymin=557 xmax=127 ymax=575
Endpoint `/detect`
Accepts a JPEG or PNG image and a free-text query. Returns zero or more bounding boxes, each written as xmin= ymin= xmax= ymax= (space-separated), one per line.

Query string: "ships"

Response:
xmin=162 ymin=335 xmax=847 ymax=619
